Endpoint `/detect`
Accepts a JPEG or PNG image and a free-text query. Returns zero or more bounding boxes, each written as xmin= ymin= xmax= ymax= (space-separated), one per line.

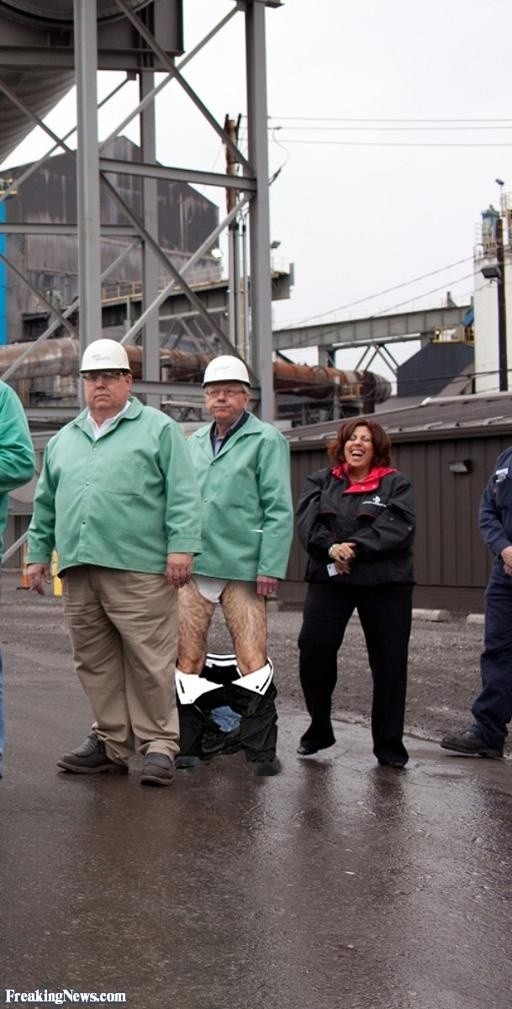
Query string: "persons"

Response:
xmin=294 ymin=419 xmax=415 ymax=770
xmin=24 ymin=339 xmax=202 ymax=785
xmin=178 ymin=355 xmax=293 ymax=774
xmin=442 ymin=446 xmax=511 ymax=759
xmin=0 ymin=378 xmax=35 ymax=780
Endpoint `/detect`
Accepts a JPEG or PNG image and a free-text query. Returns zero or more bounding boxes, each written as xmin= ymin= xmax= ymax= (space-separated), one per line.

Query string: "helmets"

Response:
xmin=201 ymin=354 xmax=249 ymax=386
xmin=80 ymin=337 xmax=133 ymax=373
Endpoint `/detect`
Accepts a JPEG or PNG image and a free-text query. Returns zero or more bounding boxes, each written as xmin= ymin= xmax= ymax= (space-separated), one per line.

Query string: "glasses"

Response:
xmin=83 ymin=372 xmax=123 ymax=381
xmin=206 ymin=388 xmax=245 ymax=398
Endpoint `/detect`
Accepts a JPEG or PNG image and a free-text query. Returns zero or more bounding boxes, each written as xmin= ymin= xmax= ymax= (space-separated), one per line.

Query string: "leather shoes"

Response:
xmin=141 ymin=753 xmax=174 ymax=786
xmin=296 ymin=734 xmax=337 ymax=756
xmin=440 ymin=725 xmax=506 ymax=760
xmin=371 ymin=746 xmax=407 ymax=769
xmin=56 ymin=734 xmax=131 ymax=775
xmin=174 ymin=752 xmax=199 ymax=769
